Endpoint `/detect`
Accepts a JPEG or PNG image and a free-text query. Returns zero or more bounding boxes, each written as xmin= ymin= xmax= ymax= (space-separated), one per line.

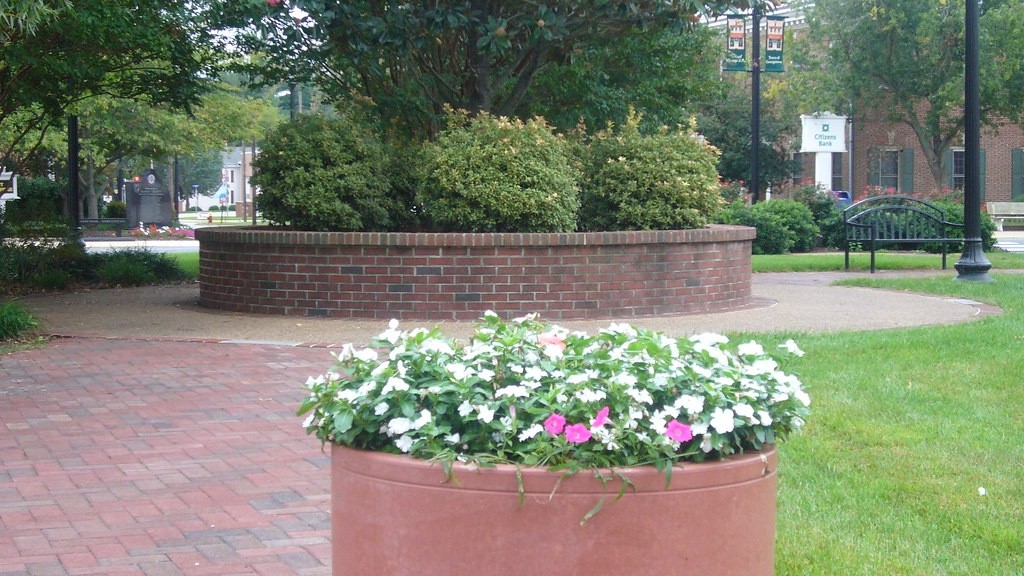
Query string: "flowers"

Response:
xmin=296 ymin=310 xmax=811 ymax=528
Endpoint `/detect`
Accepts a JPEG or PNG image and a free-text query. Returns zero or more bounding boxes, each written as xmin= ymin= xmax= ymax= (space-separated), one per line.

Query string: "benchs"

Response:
xmin=986 ymin=201 xmax=1024 ymax=231
xmin=844 ymin=195 xmax=965 ymax=273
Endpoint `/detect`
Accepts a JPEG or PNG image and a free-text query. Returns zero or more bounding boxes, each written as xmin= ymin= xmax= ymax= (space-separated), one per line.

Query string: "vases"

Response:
xmin=330 ymin=442 xmax=778 ymax=576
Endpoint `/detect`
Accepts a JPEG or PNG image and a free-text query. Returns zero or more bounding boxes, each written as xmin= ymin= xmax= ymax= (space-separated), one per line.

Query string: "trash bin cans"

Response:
xmin=830 ymin=190 xmax=852 ymax=207
